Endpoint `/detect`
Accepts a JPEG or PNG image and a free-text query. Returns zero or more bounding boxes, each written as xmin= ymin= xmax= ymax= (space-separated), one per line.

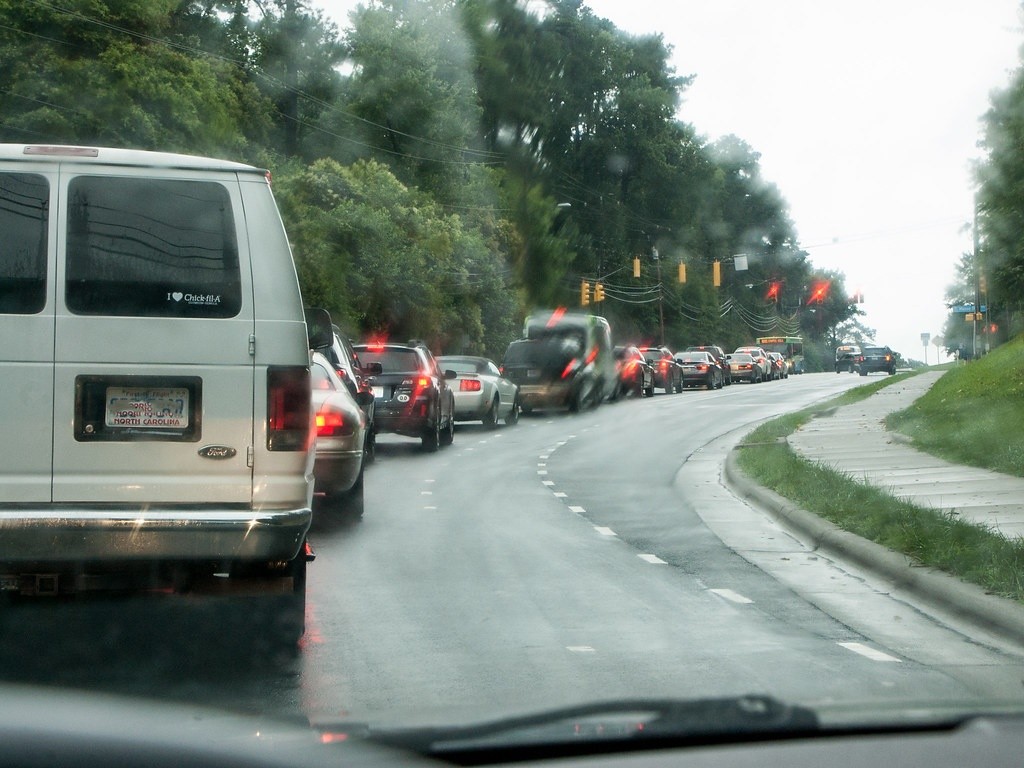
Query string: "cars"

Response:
xmin=612 ymin=345 xmax=731 ymax=398
xmin=725 ymin=345 xmax=788 ymax=383
xmin=309 ymin=320 xmax=582 ymax=518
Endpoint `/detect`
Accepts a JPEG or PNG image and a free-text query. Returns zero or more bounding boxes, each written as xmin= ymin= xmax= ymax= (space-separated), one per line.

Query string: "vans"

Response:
xmin=0 ymin=144 xmax=315 ymax=652
xmin=522 ymin=312 xmax=612 ymax=412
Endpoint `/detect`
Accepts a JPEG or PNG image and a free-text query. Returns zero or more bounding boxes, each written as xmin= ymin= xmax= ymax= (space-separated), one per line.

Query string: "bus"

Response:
xmin=756 ymin=336 xmax=805 ymax=374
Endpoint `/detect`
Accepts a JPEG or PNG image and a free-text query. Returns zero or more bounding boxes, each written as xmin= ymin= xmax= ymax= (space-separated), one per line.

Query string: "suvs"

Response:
xmin=834 ymin=345 xmax=897 ymax=376
